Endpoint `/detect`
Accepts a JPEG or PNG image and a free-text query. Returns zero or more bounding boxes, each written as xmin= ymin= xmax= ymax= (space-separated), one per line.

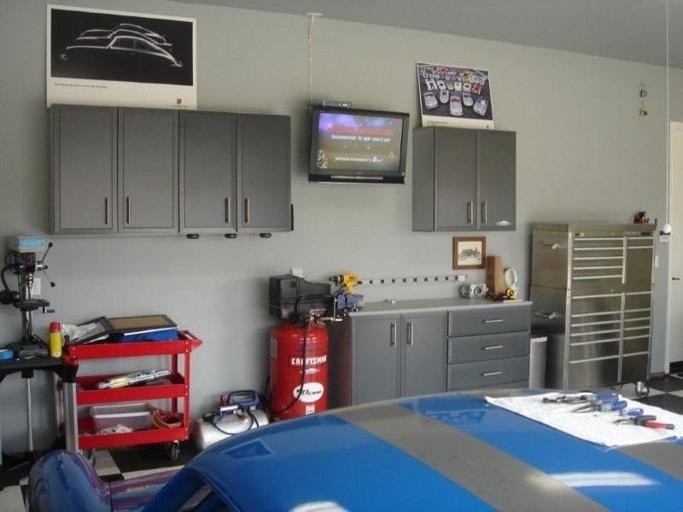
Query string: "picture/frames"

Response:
xmin=451 ymin=234 xmax=487 ymax=273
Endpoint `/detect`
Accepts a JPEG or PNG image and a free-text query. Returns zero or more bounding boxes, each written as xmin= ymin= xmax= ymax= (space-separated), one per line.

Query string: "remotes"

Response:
xmin=320 ymin=99 xmax=352 ymax=109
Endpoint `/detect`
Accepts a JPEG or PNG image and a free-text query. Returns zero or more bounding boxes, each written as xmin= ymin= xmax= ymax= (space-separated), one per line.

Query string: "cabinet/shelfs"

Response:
xmin=329 ymin=300 xmax=446 ymax=406
xmin=523 ymin=215 xmax=661 ymax=392
xmin=45 ymin=98 xmax=178 ymax=236
xmin=410 ymin=123 xmax=521 ymax=236
xmin=446 ymin=294 xmax=533 ymax=392
xmin=174 ymin=104 xmax=300 ymax=239
xmin=52 ymin=321 xmax=210 ymax=464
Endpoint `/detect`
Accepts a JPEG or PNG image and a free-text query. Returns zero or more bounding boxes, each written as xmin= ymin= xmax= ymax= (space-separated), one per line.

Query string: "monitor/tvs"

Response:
xmin=308 ymin=106 xmax=410 ymax=184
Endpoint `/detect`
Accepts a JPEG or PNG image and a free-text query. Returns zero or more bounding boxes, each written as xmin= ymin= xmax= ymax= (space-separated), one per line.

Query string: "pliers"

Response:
xmin=543 ymin=392 xmax=675 ymax=429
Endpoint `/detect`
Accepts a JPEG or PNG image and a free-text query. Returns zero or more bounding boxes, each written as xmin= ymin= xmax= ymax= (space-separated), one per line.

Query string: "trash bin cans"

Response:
xmin=529 ymin=336 xmax=549 ymax=390
